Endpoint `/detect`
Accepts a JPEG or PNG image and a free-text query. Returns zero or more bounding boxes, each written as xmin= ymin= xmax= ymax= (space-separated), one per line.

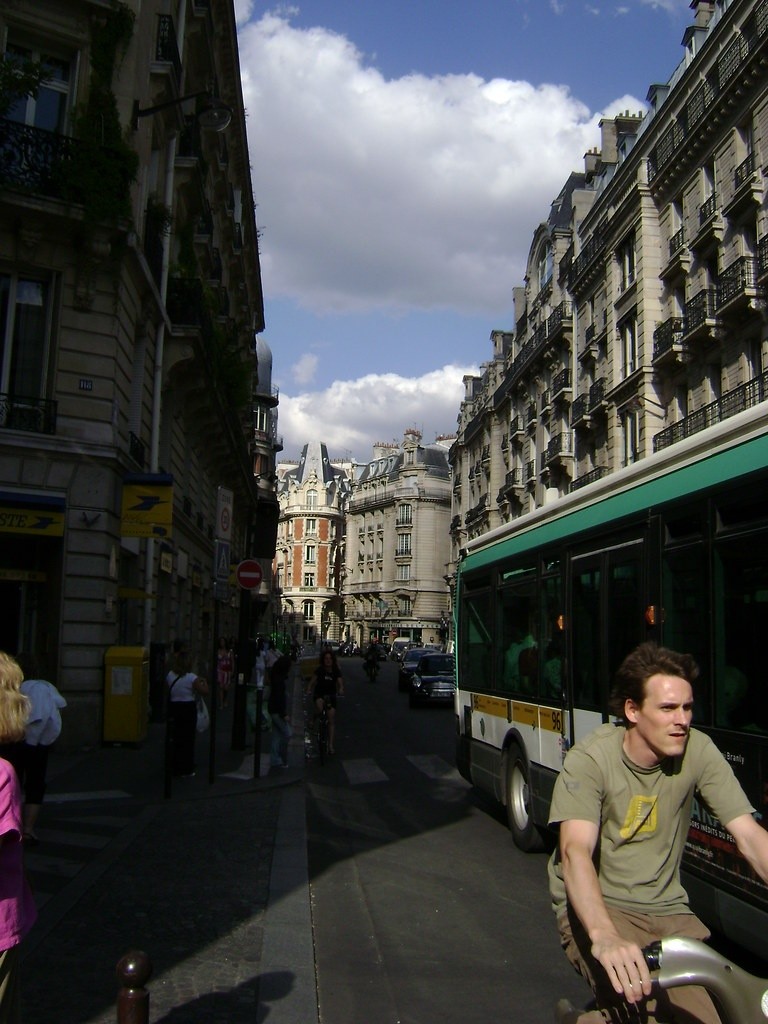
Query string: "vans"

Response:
xmin=390 ymin=635 xmax=411 ymax=660
xmin=322 ymin=640 xmax=341 ymax=653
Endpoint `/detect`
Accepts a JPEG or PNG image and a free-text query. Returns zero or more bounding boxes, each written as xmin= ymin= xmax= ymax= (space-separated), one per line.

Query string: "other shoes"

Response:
xmin=218 ymin=704 xmax=223 ymax=710
xmin=22 ymin=831 xmax=40 ymax=845
xmin=224 ymin=703 xmax=228 ymax=707
xmin=328 ymin=748 xmax=336 ymax=756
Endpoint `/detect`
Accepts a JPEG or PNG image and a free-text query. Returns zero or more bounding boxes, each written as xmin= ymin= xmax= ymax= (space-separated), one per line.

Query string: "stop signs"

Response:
xmin=237 ymin=558 xmax=264 ymax=591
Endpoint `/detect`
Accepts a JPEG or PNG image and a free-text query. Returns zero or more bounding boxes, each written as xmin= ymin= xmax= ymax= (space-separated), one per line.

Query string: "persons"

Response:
xmin=306 ymin=651 xmax=345 ymax=755
xmin=0 ymin=651 xmax=38 ymax=1024
xmin=0 ymin=680 xmax=68 ymax=840
xmin=218 ymin=634 xmax=296 ymax=721
xmin=505 ymin=608 xmax=758 ymax=731
xmin=165 ymin=648 xmax=208 ymax=777
xmin=544 ymin=641 xmax=768 ymax=1024
xmin=363 ymin=645 xmax=380 ymax=681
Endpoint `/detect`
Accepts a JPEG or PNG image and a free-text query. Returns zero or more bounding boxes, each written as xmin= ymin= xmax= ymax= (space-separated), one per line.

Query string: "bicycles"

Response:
xmin=309 ymin=691 xmax=339 ymax=766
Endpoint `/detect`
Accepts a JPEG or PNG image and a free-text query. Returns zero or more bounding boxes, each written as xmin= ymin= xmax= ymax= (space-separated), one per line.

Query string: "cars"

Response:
xmin=396 ymin=647 xmax=441 ymax=692
xmin=408 ymin=654 xmax=454 ymax=709
xmin=409 ymin=641 xmax=438 ymax=649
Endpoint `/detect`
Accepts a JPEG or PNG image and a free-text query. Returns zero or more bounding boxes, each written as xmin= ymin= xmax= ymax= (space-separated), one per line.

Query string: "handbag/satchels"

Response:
xmin=368 ymin=654 xmax=372 ymax=660
xmin=195 ymin=695 xmax=210 ymax=734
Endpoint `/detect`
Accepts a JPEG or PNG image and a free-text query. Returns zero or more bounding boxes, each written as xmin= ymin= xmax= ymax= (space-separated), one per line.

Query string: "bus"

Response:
xmin=448 ymin=395 xmax=767 ymax=970
